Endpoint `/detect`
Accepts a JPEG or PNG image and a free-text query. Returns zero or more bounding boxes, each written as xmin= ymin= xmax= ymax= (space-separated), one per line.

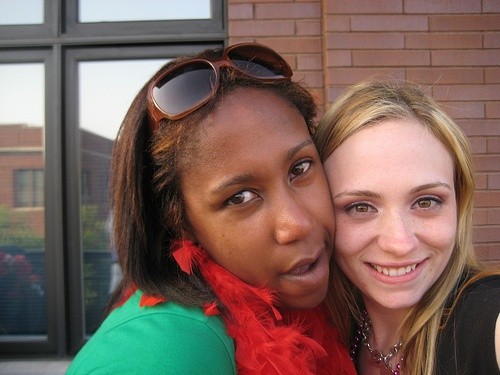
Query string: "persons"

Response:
xmin=313 ymin=78 xmax=500 ymax=375
xmin=63 ymin=47 xmax=337 ymax=375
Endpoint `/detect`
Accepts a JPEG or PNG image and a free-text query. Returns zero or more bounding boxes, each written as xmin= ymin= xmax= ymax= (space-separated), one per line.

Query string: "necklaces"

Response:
xmin=348 ymin=308 xmax=409 ymax=375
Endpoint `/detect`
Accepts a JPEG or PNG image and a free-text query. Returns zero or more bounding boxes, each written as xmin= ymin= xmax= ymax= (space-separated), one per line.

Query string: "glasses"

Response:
xmin=148 ymin=42 xmax=293 ymax=121
